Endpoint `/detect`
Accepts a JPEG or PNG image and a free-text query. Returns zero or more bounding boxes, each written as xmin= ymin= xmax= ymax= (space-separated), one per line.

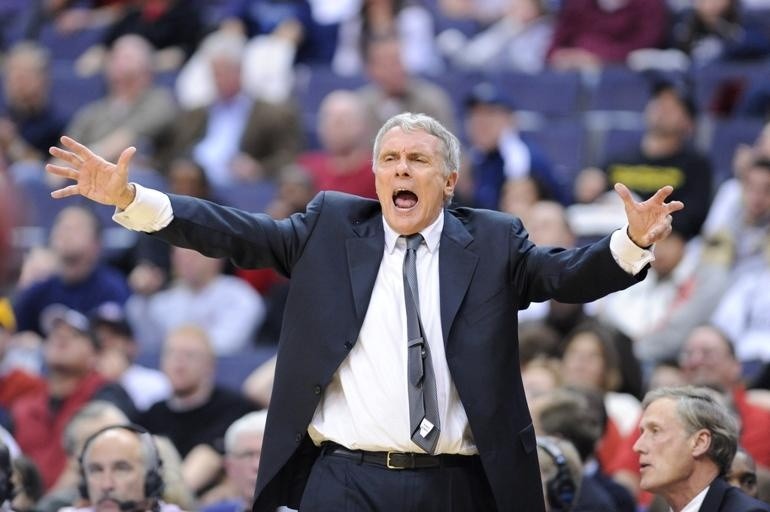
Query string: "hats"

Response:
xmin=31 ymin=296 xmax=137 ymax=348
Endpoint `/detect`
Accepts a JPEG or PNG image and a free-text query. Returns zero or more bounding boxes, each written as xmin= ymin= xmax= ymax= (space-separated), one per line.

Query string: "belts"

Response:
xmin=324 ymin=444 xmax=479 ymax=473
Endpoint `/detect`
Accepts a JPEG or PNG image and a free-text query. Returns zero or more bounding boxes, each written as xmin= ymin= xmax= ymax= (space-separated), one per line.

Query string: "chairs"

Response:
xmin=1 ymin=1 xmax=769 ymax=395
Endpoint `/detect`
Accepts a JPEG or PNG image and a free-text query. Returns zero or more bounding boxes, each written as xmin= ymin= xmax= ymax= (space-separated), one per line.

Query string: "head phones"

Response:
xmin=78 ymin=425 xmax=162 ymax=495
xmin=537 ymin=437 xmax=578 ymax=510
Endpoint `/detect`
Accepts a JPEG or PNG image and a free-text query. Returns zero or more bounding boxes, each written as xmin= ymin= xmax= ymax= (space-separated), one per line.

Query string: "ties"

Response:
xmin=398 ymin=233 xmax=442 ymax=457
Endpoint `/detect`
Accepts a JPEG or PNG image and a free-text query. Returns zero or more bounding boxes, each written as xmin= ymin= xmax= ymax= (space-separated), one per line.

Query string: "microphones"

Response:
xmin=118 ymin=498 xmax=140 ymax=509
